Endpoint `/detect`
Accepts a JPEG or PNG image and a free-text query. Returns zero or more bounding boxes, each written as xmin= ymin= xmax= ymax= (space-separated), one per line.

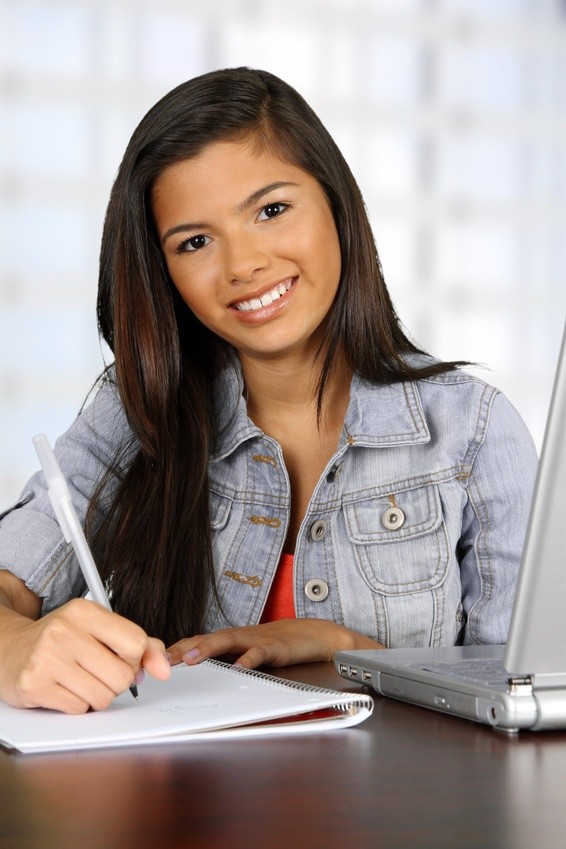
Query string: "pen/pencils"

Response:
xmin=33 ymin=432 xmax=141 ymax=703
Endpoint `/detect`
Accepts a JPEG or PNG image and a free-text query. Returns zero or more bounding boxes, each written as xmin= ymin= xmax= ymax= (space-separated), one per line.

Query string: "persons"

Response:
xmin=1 ymin=69 xmax=538 ymax=713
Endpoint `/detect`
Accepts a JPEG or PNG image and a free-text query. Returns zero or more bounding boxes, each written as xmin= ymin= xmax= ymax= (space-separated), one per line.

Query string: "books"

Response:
xmin=0 ymin=656 xmax=374 ymax=754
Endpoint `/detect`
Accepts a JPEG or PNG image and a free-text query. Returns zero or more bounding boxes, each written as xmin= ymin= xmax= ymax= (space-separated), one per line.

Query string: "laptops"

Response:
xmin=335 ymin=324 xmax=566 ymax=732
